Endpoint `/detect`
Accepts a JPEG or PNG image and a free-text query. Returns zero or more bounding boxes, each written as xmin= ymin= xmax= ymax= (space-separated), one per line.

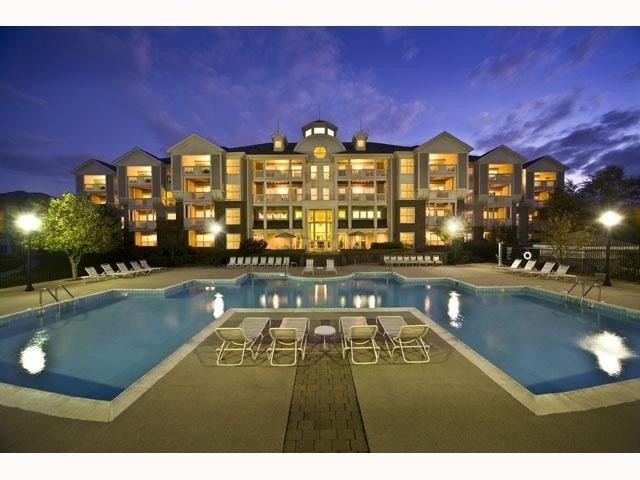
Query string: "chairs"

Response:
xmin=213 ymin=316 xmax=311 ymax=368
xmin=383 ymin=255 xmax=443 ymax=270
xmin=301 ymin=258 xmax=337 ymax=277
xmin=492 ymin=258 xmax=570 ymax=281
xmin=227 ymin=257 xmax=290 ymax=275
xmin=338 ymin=316 xmax=431 ymax=365
xmin=84 ymin=260 xmax=160 ymax=283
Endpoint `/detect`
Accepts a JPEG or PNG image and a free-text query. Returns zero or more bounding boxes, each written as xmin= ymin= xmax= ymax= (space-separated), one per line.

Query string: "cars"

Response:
xmin=147 ymin=246 xmax=193 ymax=266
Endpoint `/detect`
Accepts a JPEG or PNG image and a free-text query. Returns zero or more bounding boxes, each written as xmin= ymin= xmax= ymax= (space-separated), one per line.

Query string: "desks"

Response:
xmin=314 ymin=325 xmax=336 ymax=349
xmin=78 ymin=276 xmax=90 ymax=282
xmin=562 ymin=274 xmax=577 ymax=282
xmin=290 ymin=262 xmax=297 ymax=271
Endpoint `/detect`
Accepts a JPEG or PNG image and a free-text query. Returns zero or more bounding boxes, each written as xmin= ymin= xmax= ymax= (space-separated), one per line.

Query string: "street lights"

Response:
xmin=599 ymin=211 xmax=620 ymax=282
xmin=16 ymin=213 xmax=43 ymax=286
xmin=208 ymin=223 xmax=222 ymax=266
xmin=446 ymin=219 xmax=460 ymax=265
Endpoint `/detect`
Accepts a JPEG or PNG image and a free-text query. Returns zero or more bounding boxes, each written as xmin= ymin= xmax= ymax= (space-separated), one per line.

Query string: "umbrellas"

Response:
xmin=275 ymin=232 xmax=296 ymax=240
xmin=348 ymin=230 xmax=368 ymax=237
